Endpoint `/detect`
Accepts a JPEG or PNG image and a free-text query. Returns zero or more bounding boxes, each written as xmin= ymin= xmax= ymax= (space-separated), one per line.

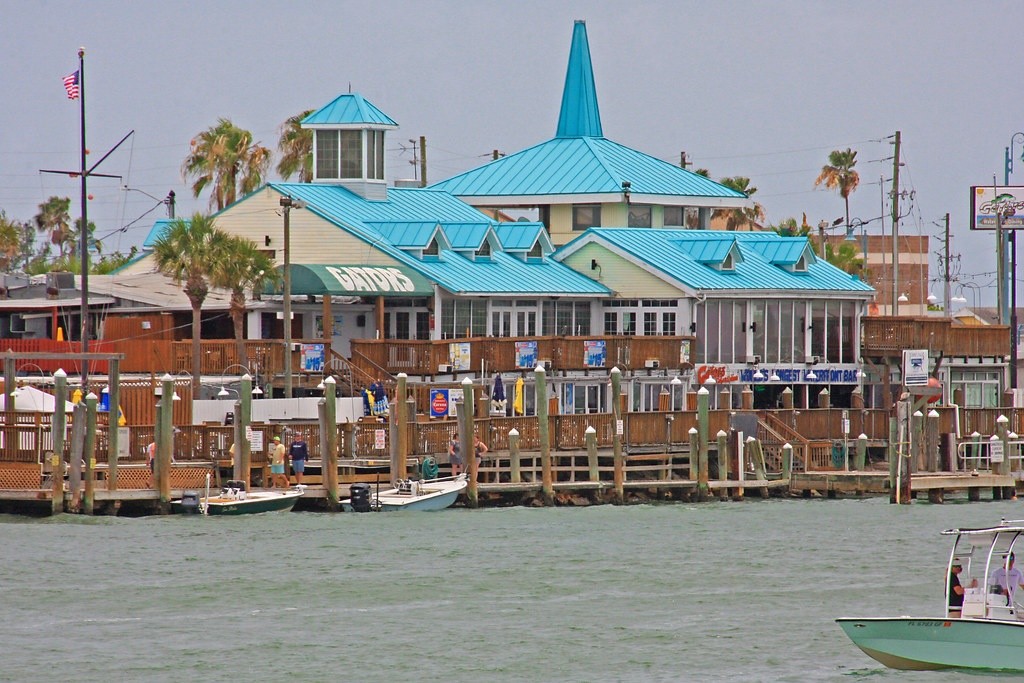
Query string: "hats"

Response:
xmin=1002 ymin=551 xmax=1014 ymax=560
xmin=274 ymin=436 xmax=280 ymax=440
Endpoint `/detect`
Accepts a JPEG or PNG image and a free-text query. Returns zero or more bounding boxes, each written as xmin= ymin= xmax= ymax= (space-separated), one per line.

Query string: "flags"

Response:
xmin=61 ymin=69 xmax=79 ymax=99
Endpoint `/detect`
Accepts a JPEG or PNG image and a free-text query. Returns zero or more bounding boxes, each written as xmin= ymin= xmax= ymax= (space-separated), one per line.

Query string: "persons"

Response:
xmin=472 ymin=434 xmax=488 ymax=481
xmin=267 ymin=436 xmax=289 ymax=489
xmin=287 ymin=432 xmax=308 ymax=495
xmin=145 ymin=438 xmax=155 ymax=489
xmin=448 ymin=433 xmax=463 ymax=477
xmin=985 ymin=551 xmax=1024 ymax=618
xmin=945 ymin=558 xmax=978 ymax=620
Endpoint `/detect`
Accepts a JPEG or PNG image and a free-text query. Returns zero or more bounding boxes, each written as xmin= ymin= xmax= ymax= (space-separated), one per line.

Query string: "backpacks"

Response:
xmin=479 ymin=442 xmax=484 ymax=460
xmin=448 ymin=440 xmax=455 ymax=456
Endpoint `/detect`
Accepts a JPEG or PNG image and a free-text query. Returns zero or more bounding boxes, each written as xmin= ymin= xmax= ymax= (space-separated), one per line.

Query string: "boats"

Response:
xmin=340 ymin=472 xmax=468 ymax=512
xmin=835 ymin=517 xmax=1024 ymax=670
xmin=169 ymin=481 xmax=304 ymax=514
